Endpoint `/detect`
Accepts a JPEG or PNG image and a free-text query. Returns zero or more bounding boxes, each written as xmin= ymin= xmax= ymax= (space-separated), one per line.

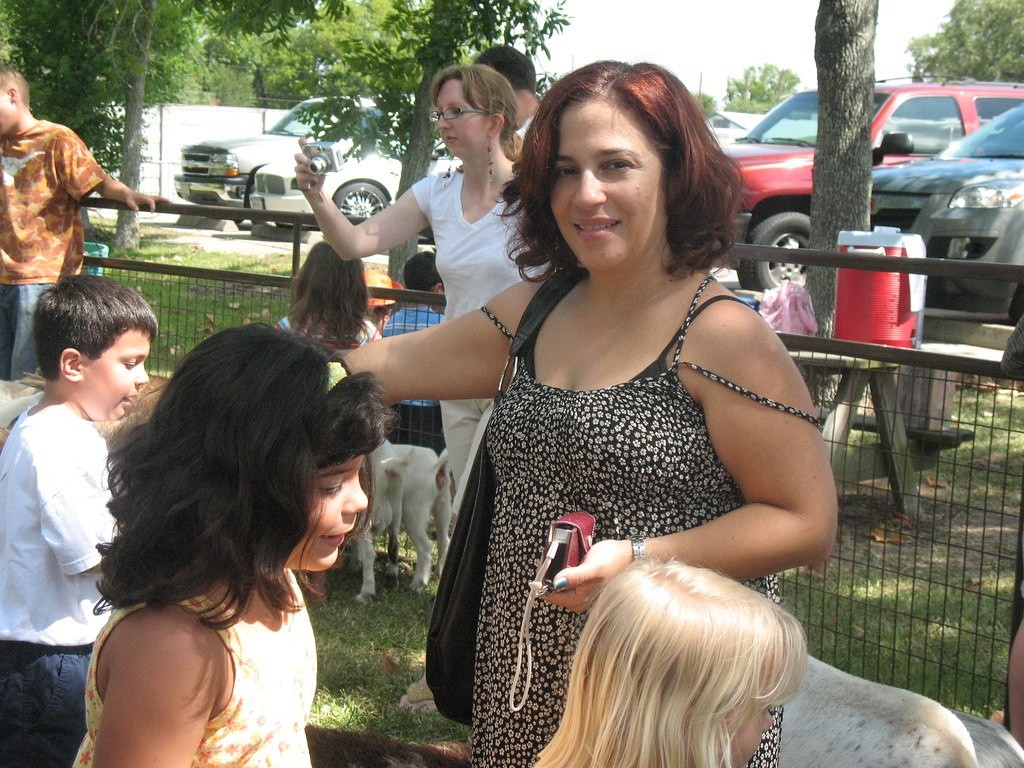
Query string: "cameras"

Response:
xmin=300 ymin=141 xmax=344 ymax=174
xmin=544 ymin=523 xmax=573 ymax=583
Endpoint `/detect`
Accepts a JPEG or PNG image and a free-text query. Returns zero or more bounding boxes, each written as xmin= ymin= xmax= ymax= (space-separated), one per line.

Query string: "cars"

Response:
xmin=866 ymin=103 xmax=1024 ymax=265
xmin=248 ymin=101 xmax=465 ymax=229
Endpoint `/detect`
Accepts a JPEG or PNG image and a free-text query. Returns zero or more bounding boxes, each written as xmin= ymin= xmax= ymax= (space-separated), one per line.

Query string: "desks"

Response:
xmin=780 ymin=347 xmax=942 ymax=484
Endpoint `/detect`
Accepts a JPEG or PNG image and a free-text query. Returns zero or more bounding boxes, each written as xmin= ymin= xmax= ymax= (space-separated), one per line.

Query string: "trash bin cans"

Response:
xmin=81 ymin=242 xmax=109 ymax=276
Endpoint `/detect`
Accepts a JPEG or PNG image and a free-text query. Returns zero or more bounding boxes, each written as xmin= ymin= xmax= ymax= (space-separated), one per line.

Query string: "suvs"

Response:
xmin=171 ymin=96 xmax=377 ymax=229
xmin=713 ymin=79 xmax=1024 ymax=298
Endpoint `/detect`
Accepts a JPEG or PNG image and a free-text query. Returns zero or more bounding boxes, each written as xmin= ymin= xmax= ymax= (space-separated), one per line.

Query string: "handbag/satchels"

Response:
xmin=426 ymin=270 xmax=582 ymax=727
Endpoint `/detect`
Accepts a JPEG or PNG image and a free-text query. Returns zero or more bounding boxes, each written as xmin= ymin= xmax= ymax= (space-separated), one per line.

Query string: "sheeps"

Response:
xmin=350 ymin=438 xmax=452 ymax=603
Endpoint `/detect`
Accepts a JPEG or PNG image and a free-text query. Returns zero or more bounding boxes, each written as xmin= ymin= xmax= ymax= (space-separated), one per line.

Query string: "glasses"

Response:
xmin=429 ymin=107 xmax=490 ymax=122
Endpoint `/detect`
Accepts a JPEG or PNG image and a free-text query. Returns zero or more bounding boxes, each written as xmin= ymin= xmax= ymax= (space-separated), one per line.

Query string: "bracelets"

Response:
xmin=630 ymin=535 xmax=646 ymax=561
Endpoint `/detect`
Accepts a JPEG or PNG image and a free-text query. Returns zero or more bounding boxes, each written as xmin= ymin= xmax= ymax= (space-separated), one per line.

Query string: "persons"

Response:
xmin=342 ymin=60 xmax=839 ymax=768
xmin=71 ymin=325 xmax=401 ymax=768
xmin=1001 ymin=316 xmax=1024 ymax=752
xmin=0 ymin=274 xmax=158 ymax=768
xmin=0 ymin=68 xmax=173 ymax=381
xmin=274 ymin=47 xmax=562 ymax=714
xmin=534 ymin=557 xmax=807 ymax=768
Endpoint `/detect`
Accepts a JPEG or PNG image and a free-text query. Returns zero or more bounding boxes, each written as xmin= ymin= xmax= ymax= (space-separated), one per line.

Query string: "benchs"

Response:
xmin=804 ymin=404 xmax=974 ymax=482
